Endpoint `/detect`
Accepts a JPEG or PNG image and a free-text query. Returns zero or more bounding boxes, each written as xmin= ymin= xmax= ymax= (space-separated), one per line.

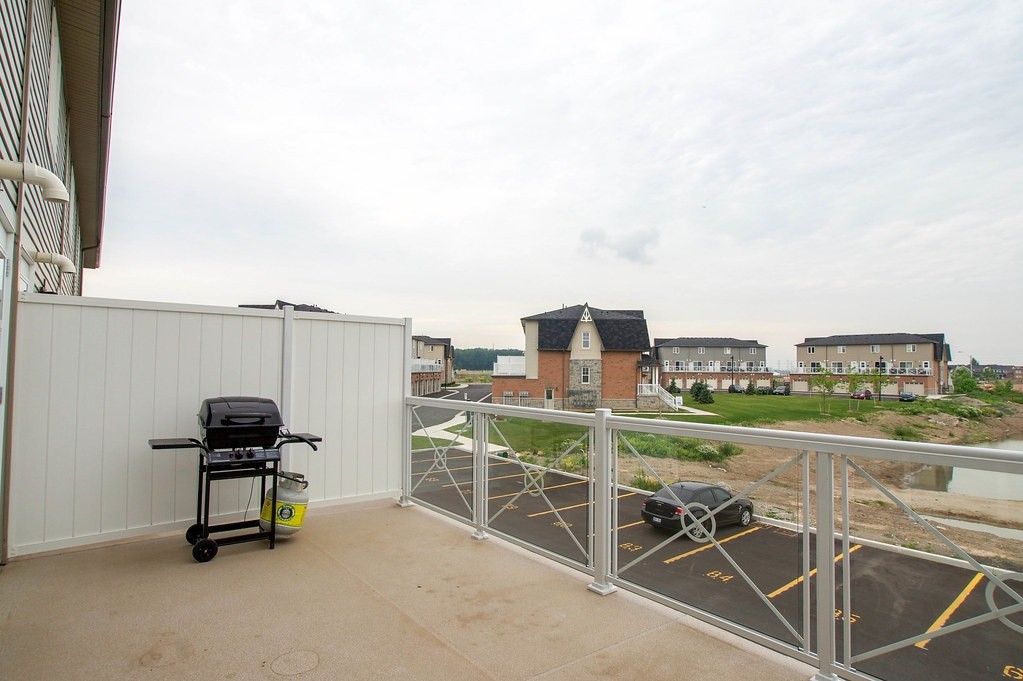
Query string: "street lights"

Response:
xmin=879 ymin=356 xmax=884 ymax=401
xmin=958 ymin=351 xmax=972 ymax=376
xmin=730 ymin=355 xmax=735 ymax=393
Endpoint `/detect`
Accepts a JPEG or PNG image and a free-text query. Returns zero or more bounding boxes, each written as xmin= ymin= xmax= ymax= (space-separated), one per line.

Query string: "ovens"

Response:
xmin=195 ymin=395 xmax=285 ymax=449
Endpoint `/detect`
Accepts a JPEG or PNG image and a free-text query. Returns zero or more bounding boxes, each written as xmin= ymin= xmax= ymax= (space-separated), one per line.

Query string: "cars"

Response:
xmin=706 ymin=384 xmax=714 ymax=393
xmin=758 ymin=386 xmax=773 ymax=396
xmin=665 ymin=385 xmax=681 ymax=394
xmin=850 ymin=389 xmax=872 ymax=400
xmin=773 ymin=386 xmax=790 ymax=396
xmin=729 ymin=384 xmax=745 ymax=393
xmin=640 ymin=481 xmax=754 ymax=539
xmin=898 ymin=392 xmax=916 ymax=402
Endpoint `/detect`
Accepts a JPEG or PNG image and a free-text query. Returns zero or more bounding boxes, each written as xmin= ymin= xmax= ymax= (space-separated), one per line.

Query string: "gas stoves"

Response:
xmin=200 ymin=445 xmax=281 ymax=464
